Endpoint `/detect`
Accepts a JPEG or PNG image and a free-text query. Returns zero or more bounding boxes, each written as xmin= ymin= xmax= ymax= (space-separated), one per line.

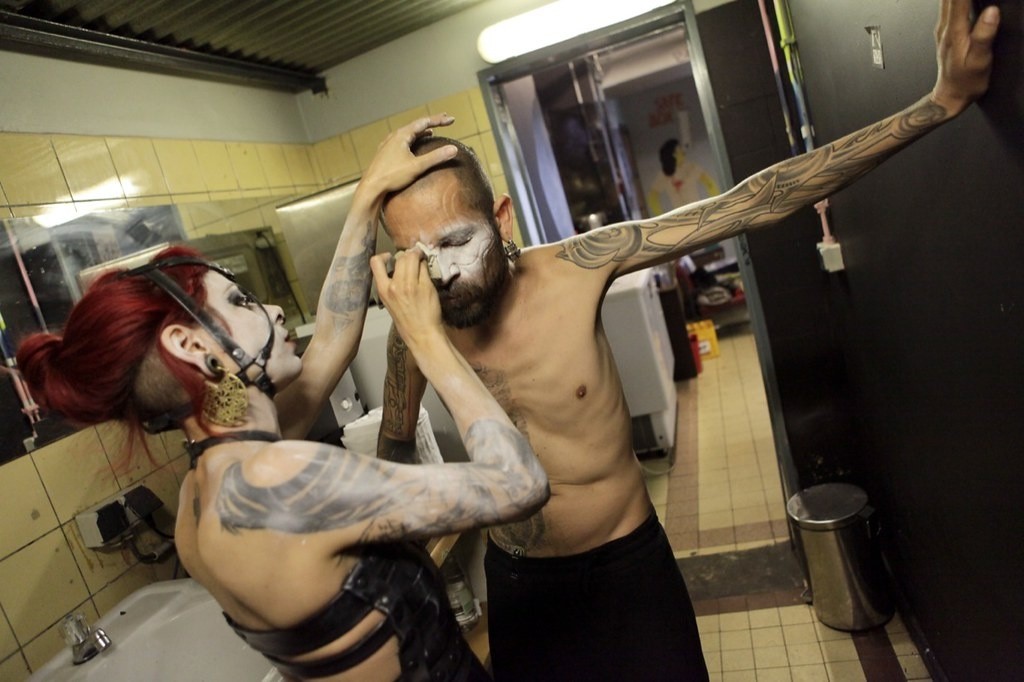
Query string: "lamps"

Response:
xmin=477 ymin=0 xmax=676 ymax=64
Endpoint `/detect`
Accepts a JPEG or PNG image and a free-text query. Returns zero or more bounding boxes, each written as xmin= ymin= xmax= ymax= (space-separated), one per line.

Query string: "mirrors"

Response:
xmin=0 ymin=193 xmax=312 ymax=476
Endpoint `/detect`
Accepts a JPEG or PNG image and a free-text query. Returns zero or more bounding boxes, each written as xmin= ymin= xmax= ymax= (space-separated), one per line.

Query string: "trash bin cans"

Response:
xmin=784 ymin=482 xmax=897 ymax=634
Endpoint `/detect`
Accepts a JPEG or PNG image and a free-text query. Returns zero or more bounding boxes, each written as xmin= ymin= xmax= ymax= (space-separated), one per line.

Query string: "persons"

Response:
xmin=375 ymin=0 xmax=1003 ymax=682
xmin=11 ymin=109 xmax=555 ymax=680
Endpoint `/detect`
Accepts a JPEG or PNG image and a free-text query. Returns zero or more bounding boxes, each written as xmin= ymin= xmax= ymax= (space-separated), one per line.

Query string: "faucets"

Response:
xmin=55 ymin=612 xmax=111 ymax=664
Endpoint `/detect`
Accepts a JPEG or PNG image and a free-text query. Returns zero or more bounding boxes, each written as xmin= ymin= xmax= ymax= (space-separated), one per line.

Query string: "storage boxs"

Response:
xmin=689 ymin=334 xmax=704 ymax=375
xmin=686 ymin=316 xmax=721 ymax=359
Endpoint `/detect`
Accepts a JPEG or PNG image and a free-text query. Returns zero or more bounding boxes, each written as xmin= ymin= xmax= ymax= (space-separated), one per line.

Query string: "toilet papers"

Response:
xmin=339 ymin=399 xmax=445 ymax=471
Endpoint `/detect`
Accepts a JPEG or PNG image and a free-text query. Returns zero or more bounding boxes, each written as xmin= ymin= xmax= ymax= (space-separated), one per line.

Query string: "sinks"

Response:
xmin=22 ymin=576 xmax=281 ymax=682
